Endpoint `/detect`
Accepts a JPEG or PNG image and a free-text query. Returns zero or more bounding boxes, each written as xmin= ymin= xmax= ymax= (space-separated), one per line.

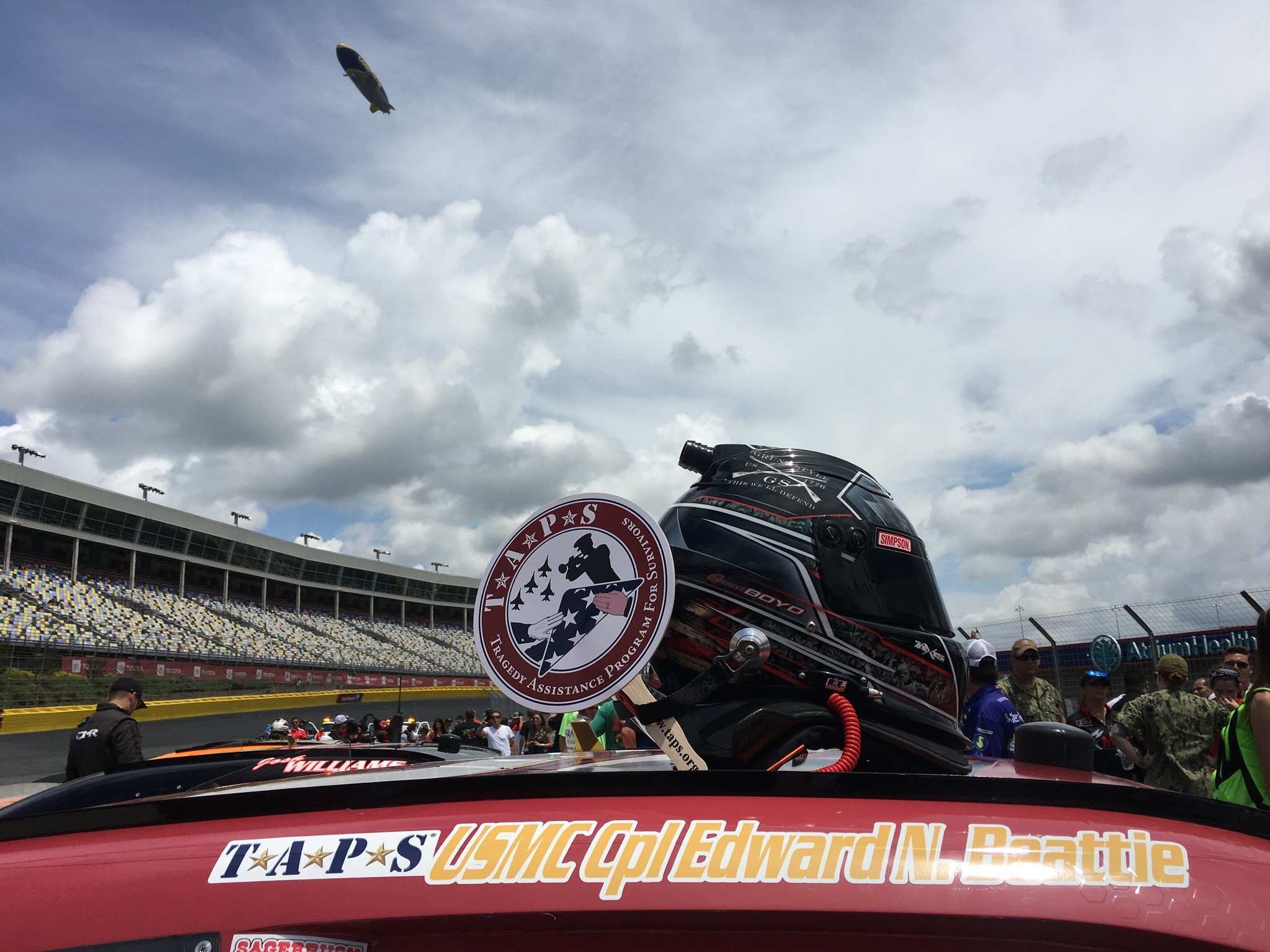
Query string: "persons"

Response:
xmin=66 ymin=677 xmax=148 ymax=780
xmin=0 ymin=709 xmax=4 ymax=729
xmin=960 ymin=638 xmax=1270 ymax=815
xmin=287 ymin=700 xmax=659 ymax=762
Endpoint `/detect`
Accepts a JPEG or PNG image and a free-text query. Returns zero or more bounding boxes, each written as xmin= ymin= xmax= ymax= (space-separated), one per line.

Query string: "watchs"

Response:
xmin=481 ymin=725 xmax=486 ymax=729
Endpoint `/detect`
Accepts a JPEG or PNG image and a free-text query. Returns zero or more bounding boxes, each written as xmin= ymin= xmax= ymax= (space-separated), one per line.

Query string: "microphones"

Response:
xmin=511 ymin=720 xmax=514 ymax=721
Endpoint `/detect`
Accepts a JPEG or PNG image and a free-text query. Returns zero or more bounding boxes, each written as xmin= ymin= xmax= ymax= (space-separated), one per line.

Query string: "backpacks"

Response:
xmin=1213 ymin=686 xmax=1270 ymax=810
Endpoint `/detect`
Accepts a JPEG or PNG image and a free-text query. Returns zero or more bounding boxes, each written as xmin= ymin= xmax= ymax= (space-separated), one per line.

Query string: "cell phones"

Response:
xmin=484 ymin=714 xmax=492 ymax=723
xmin=531 ymin=741 xmax=534 ymax=745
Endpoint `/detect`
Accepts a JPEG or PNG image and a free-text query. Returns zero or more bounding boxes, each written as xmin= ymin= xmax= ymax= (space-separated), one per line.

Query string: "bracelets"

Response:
xmin=541 ymin=743 xmax=545 ymax=747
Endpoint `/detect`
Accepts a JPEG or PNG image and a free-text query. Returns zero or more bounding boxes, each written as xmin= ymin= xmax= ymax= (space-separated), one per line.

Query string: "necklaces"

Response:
xmin=1089 ymin=710 xmax=1105 ymax=717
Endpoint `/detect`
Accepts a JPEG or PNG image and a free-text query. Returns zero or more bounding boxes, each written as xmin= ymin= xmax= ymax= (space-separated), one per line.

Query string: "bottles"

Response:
xmin=1116 ymin=744 xmax=1136 ymax=771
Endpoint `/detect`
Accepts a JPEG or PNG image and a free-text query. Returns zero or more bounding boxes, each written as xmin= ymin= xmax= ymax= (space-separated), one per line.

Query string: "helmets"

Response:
xmin=650 ymin=441 xmax=975 ymax=772
xmin=272 ymin=718 xmax=289 ymax=732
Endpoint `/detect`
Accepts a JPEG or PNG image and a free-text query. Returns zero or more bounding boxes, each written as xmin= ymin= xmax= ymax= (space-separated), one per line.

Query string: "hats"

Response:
xmin=962 ymin=638 xmax=997 ymax=667
xmin=1157 ymin=654 xmax=1188 ymax=676
xmin=527 ymin=708 xmax=535 ymax=713
xmin=1209 ymin=668 xmax=1238 ymax=680
xmin=483 ymin=708 xmax=493 ymax=716
xmin=110 ymin=678 xmax=147 ymax=711
xmin=343 ymin=714 xmax=355 ymax=721
xmin=445 ymin=718 xmax=453 ymax=723
xmin=1080 ymin=669 xmax=1111 ymax=686
xmin=512 ymin=712 xmax=522 ymax=718
xmin=332 ymin=714 xmax=348 ymax=727
xmin=1012 ymin=638 xmax=1038 ymax=658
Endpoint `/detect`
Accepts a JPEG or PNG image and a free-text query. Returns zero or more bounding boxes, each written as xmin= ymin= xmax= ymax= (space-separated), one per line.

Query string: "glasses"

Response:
xmin=533 ymin=718 xmax=539 ymax=720
xmin=1154 ymin=670 xmax=1159 ymax=681
xmin=1223 ymin=661 xmax=1249 ymax=669
xmin=513 ymin=718 xmax=518 ymax=720
xmin=131 ymin=689 xmax=141 ymax=707
xmin=1088 ymin=670 xmax=1107 ymax=677
xmin=589 ymin=705 xmax=598 ymax=710
xmin=491 ymin=716 xmax=501 ymax=719
xmin=0 ymin=718 xmax=3 ymax=720
xmin=1015 ymin=654 xmax=1039 ymax=661
xmin=292 ymin=721 xmax=299 ymax=724
